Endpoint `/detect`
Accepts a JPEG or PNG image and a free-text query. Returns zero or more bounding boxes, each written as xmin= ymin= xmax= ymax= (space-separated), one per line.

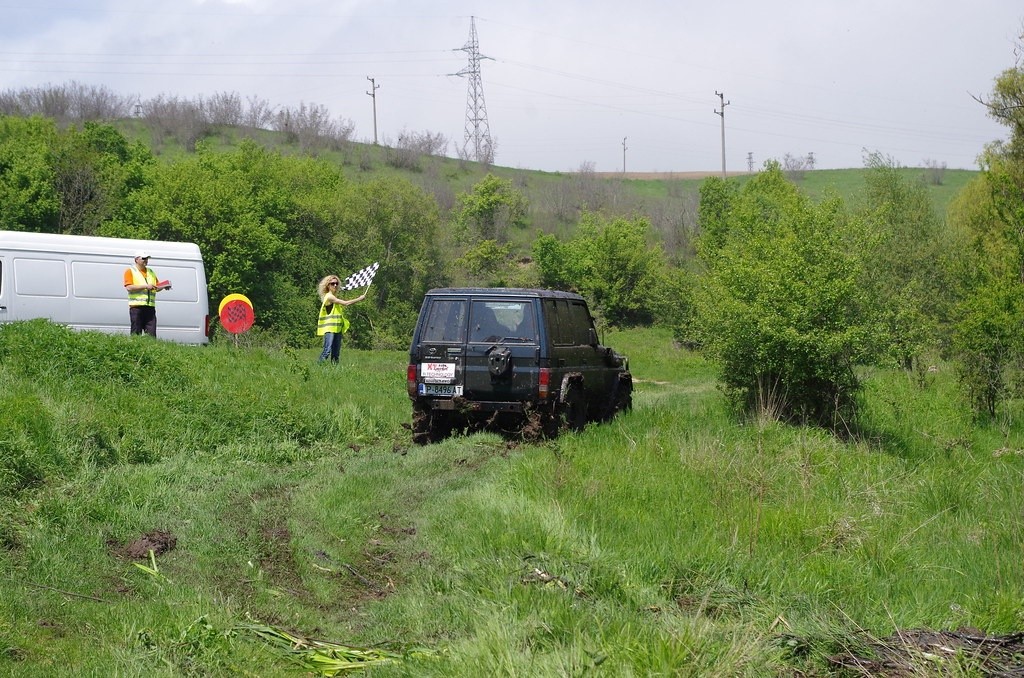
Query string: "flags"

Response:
xmin=341 ymin=262 xmax=379 ymax=291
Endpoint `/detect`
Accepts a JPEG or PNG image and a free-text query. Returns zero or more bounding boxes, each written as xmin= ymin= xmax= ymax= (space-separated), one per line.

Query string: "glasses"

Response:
xmin=139 ymin=257 xmax=148 ymax=260
xmin=329 ymin=282 xmax=339 ymax=286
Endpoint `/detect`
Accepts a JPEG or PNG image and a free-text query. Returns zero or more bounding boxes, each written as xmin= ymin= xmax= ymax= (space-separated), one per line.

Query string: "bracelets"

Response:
xmin=146 ymin=285 xmax=147 ymax=288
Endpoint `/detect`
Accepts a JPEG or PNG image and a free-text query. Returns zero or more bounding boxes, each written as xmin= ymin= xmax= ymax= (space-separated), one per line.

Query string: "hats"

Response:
xmin=134 ymin=252 xmax=151 ymax=261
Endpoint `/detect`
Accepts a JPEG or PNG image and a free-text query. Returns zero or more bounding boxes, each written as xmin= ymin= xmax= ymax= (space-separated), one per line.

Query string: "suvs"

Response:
xmin=409 ymin=286 xmax=633 ymax=443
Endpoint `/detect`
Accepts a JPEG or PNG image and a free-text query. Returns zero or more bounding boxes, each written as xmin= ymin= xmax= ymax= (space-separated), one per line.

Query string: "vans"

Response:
xmin=0 ymin=229 xmax=210 ymax=346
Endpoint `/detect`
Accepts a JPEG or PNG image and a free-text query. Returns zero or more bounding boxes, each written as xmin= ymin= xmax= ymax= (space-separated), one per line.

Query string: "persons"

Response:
xmin=315 ymin=275 xmax=366 ymax=364
xmin=122 ymin=250 xmax=160 ymax=339
